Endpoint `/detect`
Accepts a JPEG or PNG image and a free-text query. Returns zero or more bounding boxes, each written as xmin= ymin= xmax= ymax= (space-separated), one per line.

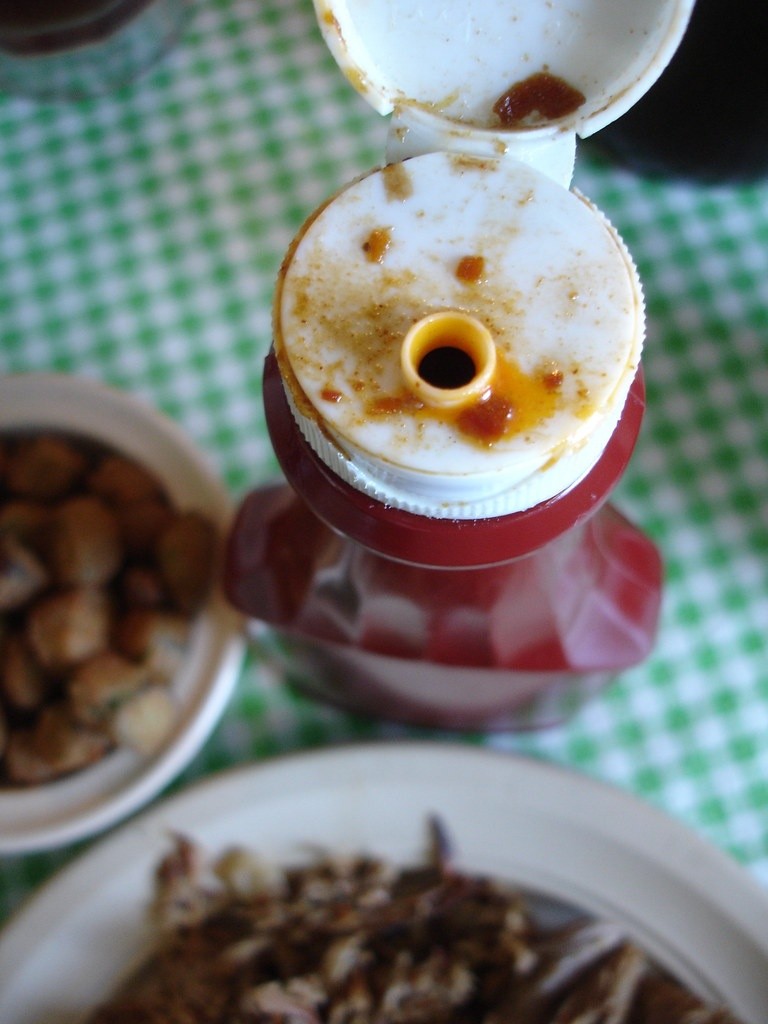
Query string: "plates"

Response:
xmin=0 ymin=737 xmax=768 ymax=1024
xmin=0 ymin=374 xmax=249 ymax=856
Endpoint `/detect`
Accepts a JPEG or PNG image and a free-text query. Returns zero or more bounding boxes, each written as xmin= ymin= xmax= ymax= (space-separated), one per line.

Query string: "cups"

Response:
xmin=0 ymin=0 xmax=193 ymax=102
